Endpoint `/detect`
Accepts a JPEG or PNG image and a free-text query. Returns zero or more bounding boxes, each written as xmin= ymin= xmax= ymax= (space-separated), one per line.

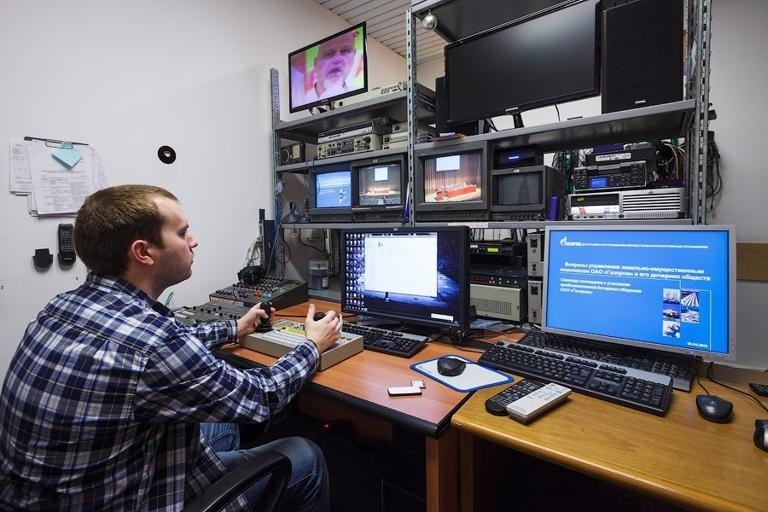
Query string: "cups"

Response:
xmin=307 ymin=260 xmax=330 ymax=290
xmin=549 ymin=196 xmax=563 ymax=221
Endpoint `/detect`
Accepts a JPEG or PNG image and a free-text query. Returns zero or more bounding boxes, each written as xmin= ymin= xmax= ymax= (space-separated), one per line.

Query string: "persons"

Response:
xmin=0 ymin=183 xmax=344 ymax=512
xmin=306 ymin=31 xmax=355 ymax=103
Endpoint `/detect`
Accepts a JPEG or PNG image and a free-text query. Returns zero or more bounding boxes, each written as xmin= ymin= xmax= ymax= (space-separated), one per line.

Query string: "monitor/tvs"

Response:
xmin=490 ymin=164 xmax=564 ymax=220
xmin=414 ymin=140 xmax=494 ymax=221
xmin=349 ymin=154 xmax=408 ymax=223
xmin=443 ymin=0 xmax=600 ymax=130
xmin=288 ymin=20 xmax=368 ymax=113
xmin=541 ymin=222 xmax=737 ymax=362
xmin=339 ymin=225 xmax=471 ymax=340
xmin=308 ymin=162 xmax=352 ymax=223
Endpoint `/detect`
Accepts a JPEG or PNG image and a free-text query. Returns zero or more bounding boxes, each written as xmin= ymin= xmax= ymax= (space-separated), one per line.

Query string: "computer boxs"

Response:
xmin=259 ymin=219 xmax=285 ymax=278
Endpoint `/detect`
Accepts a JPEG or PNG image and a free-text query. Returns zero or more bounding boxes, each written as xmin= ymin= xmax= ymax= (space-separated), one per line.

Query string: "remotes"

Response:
xmin=505 ymin=381 xmax=572 ymax=424
xmin=484 ymin=377 xmax=545 ymax=416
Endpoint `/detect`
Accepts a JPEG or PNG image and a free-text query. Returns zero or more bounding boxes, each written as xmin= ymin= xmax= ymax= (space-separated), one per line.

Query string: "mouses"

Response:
xmin=753 ymin=419 xmax=768 ymax=451
xmin=438 ymin=356 xmax=466 ymax=376
xmin=696 ymin=394 xmax=733 ymax=422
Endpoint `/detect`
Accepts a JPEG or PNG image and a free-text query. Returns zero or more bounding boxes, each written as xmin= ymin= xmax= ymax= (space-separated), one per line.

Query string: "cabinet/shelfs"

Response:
xmin=271 ymin=0 xmax=711 ymax=326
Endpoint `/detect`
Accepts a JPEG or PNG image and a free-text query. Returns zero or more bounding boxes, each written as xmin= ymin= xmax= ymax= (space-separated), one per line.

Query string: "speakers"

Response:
xmin=434 ymin=75 xmax=493 ymax=136
xmin=600 ymin=0 xmax=684 ymax=115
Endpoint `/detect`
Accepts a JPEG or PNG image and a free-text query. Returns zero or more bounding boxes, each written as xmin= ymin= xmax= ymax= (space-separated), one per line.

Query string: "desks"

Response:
xmin=451 ymin=361 xmax=767 ymax=512
xmin=212 ymin=298 xmax=528 ymax=512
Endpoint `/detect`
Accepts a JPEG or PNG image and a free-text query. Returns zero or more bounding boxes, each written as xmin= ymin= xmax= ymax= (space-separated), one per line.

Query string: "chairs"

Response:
xmin=184 ymin=451 xmax=293 ymax=512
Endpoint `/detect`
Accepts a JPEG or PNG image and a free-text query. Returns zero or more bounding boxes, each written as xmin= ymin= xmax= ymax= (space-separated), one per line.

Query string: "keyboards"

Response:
xmin=478 ymin=339 xmax=674 ymax=416
xmin=516 ymin=330 xmax=697 ymax=393
xmin=341 ymin=321 xmax=428 ymax=359
